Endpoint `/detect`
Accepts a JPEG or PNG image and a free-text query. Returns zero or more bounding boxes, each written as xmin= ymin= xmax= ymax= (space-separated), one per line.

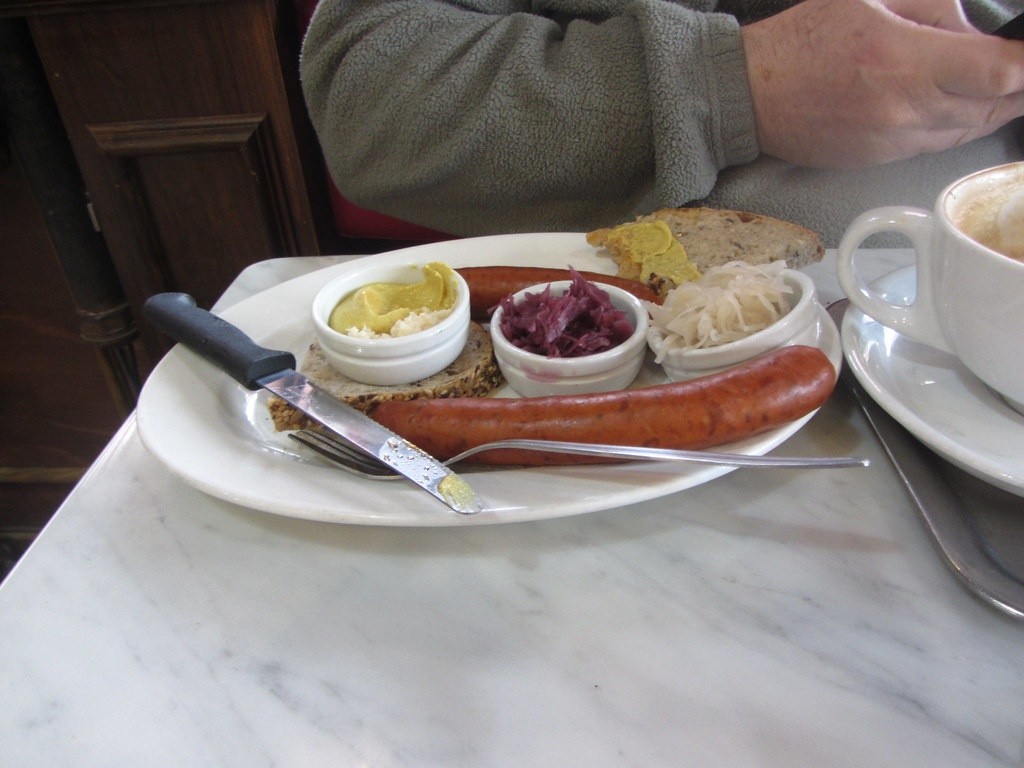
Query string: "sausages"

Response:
xmin=370 ymin=343 xmax=836 ymax=467
xmin=450 ymin=265 xmax=662 ymax=324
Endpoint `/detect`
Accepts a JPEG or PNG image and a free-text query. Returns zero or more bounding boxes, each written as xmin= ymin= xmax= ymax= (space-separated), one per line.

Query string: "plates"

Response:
xmin=841 ymin=260 xmax=1024 ymax=498
xmin=135 ymin=230 xmax=841 ymax=526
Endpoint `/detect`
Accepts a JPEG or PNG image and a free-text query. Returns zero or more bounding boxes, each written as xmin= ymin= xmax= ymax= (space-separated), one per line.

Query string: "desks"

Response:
xmin=0 ymin=250 xmax=1024 ymax=767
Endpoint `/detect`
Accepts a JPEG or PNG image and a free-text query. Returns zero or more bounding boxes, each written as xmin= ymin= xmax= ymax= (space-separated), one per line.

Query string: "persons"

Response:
xmin=300 ymin=0 xmax=1024 ymax=235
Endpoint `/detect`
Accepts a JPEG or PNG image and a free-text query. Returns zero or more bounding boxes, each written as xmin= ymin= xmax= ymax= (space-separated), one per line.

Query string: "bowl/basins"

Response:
xmin=488 ymin=280 xmax=649 ymax=397
xmin=311 ymin=264 xmax=471 ymax=385
xmin=645 ymin=263 xmax=821 ymax=382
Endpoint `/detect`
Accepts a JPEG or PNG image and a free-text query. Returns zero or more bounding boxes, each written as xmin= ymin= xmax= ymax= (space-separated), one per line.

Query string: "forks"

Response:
xmin=286 ymin=428 xmax=872 ymax=480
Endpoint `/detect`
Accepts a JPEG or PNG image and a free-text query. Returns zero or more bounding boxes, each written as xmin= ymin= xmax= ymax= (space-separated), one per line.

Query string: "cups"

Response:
xmin=838 ymin=161 xmax=1024 ymax=419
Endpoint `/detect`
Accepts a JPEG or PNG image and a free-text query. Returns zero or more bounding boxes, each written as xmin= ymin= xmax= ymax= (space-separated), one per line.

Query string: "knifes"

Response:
xmin=144 ymin=291 xmax=486 ymax=516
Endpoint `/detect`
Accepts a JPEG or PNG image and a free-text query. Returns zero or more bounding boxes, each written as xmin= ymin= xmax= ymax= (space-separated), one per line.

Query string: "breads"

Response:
xmin=265 ymin=321 xmax=500 ymax=432
xmin=582 ymin=208 xmax=826 ymax=298
